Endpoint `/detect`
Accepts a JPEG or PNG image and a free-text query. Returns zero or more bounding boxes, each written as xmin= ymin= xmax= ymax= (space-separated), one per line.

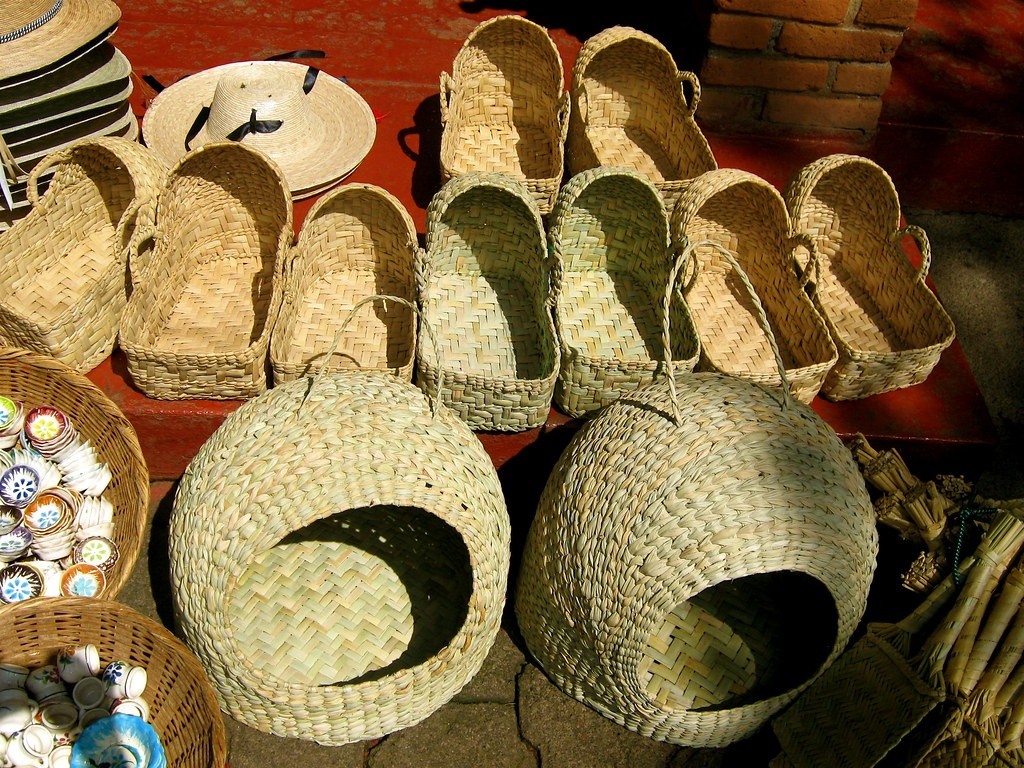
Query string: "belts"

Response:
xmin=670 ymin=167 xmax=840 ymax=407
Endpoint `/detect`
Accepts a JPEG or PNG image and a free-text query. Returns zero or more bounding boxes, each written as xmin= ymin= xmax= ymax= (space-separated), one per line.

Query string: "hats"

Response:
xmin=520 ymin=235 xmax=884 ymax=750
xmin=172 ymin=374 xmax=512 ymax=746
xmin=140 ymin=60 xmax=380 ymax=201
xmin=0 ymin=0 xmax=139 ymax=232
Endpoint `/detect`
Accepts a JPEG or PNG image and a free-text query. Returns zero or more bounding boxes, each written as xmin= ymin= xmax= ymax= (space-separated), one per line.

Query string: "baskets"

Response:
xmin=772 ymin=160 xmax=959 ymax=401
xmin=545 ymin=165 xmax=702 ymax=419
xmin=268 ymin=180 xmax=418 ymax=388
xmin=0 ymin=595 xmax=228 ymax=768
xmin=118 ymin=140 xmax=297 ymax=400
xmin=0 ymin=136 xmax=171 ymax=378
xmin=566 ymin=25 xmax=720 ymax=212
xmin=437 ymin=13 xmax=570 ymax=215
xmin=0 ymin=343 xmax=151 ymax=611
xmin=414 ymin=173 xmax=560 ymax=433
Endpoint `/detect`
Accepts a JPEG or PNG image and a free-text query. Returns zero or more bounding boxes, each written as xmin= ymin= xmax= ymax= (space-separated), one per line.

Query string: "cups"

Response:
xmin=0 ymin=643 xmax=150 ymax=768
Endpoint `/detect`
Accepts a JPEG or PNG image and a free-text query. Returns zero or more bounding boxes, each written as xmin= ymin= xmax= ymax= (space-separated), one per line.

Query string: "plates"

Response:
xmin=70 ymin=713 xmax=168 ymax=768
xmin=0 ymin=394 xmax=120 ymax=605
